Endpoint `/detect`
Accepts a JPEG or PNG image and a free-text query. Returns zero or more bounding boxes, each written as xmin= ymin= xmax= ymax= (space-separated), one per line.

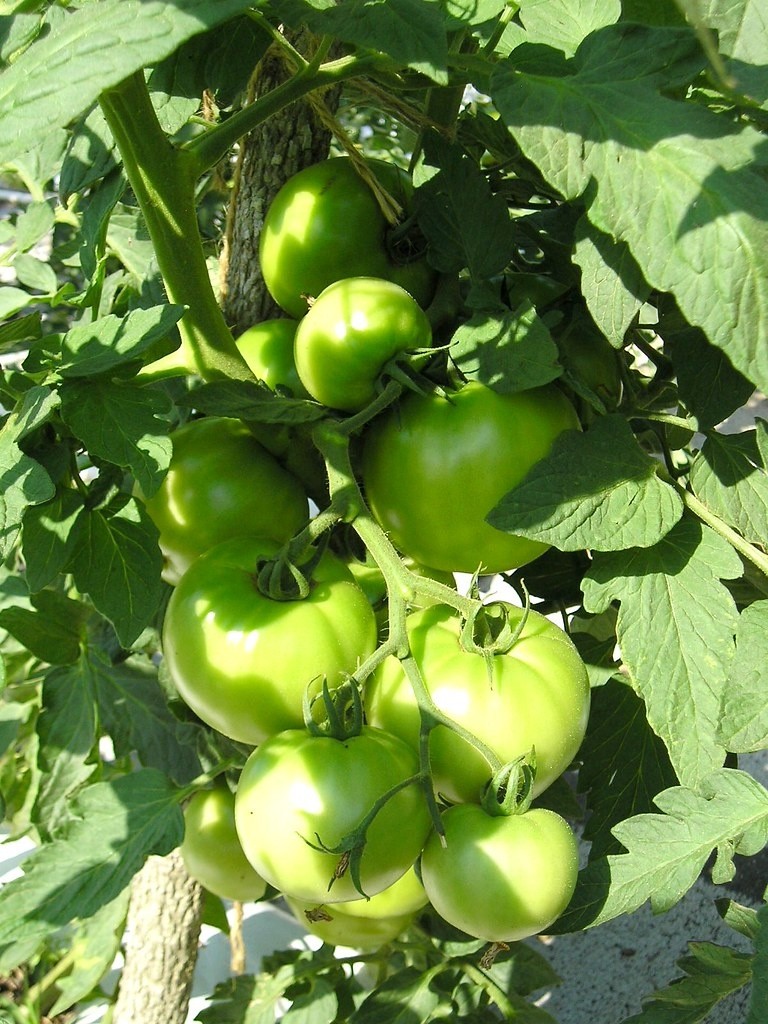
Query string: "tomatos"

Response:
xmin=293 ymin=275 xmax=432 ymax=409
xmin=233 ymin=724 xmax=433 ymax=905
xmin=326 ymin=864 xmax=429 ymax=920
xmin=131 ymin=414 xmax=309 ymax=588
xmin=283 ymin=894 xmax=411 ymax=948
xmin=179 ymin=774 xmax=285 ymax=903
xmin=333 ymin=545 xmax=458 ymax=644
xmin=357 ymin=367 xmax=583 ymax=576
xmin=163 ymin=536 xmax=378 ymax=745
xmin=421 ymin=803 xmax=578 ymax=942
xmin=259 ymin=157 xmax=437 ymax=319
xmin=362 ymin=599 xmax=590 ymax=805
xmin=235 ymin=319 xmax=313 ymax=398
